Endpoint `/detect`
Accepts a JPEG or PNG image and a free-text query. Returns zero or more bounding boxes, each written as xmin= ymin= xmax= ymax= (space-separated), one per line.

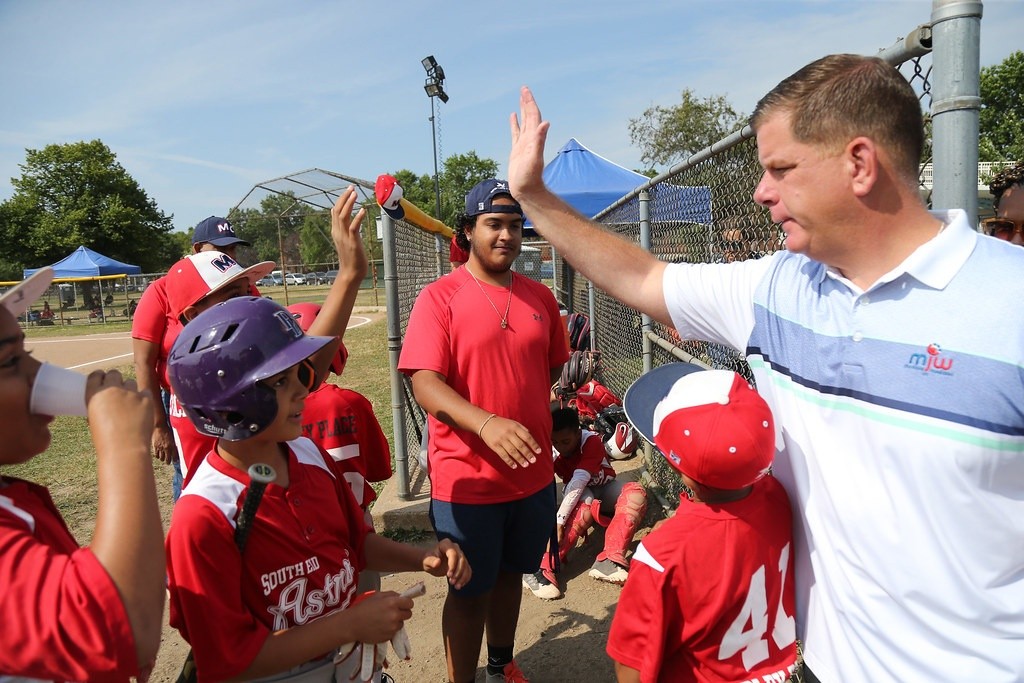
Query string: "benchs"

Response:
xmin=56 ymin=314 xmax=127 ymax=324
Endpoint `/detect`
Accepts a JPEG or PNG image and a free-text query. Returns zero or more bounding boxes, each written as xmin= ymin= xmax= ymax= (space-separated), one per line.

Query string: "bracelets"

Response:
xmin=478 ymin=413 xmax=496 ymax=440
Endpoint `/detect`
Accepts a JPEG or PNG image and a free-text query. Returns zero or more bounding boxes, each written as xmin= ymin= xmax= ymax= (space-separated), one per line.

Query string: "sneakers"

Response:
xmin=589 ymin=558 xmax=630 ymax=587
xmin=522 ymin=569 xmax=561 ymax=601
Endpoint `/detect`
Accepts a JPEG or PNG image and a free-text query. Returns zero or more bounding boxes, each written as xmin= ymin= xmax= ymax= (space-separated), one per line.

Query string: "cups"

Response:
xmin=29 ymin=361 xmax=90 ymax=418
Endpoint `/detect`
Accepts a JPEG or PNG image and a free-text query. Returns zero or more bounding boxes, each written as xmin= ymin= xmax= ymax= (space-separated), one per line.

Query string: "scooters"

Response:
xmin=123 ymin=299 xmax=138 ymax=317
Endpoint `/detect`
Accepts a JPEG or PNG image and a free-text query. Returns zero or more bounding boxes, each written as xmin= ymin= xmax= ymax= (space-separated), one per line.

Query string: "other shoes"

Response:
xmin=486 ymin=661 xmax=528 ymax=683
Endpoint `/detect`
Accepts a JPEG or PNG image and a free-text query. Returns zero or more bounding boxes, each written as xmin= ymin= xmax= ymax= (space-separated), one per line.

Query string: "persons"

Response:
xmin=93 ymin=291 xmax=101 ymax=303
xmin=41 ymin=300 xmax=50 ymax=313
xmin=166 ymin=294 xmax=472 ymax=683
xmin=653 ymin=215 xmax=786 ymax=382
xmin=508 ymin=53 xmax=1024 ymax=683
xmin=0 ymin=266 xmax=168 ymax=683
xmin=63 ymin=296 xmax=74 ymax=308
xmin=522 ymin=406 xmax=647 ymax=600
xmin=104 ymin=291 xmax=112 ymax=307
xmin=165 ymin=184 xmax=366 ymax=491
xmin=397 ymin=179 xmax=561 ymax=683
xmin=131 ymin=214 xmax=261 ymax=506
xmin=980 ymin=158 xmax=1024 ymax=246
xmin=87 ymin=300 xmax=103 ymax=324
xmin=604 ymin=360 xmax=799 ymax=683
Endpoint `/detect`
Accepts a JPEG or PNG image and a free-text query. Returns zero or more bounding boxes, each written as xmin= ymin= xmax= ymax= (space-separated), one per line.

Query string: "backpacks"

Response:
xmin=553 ymin=348 xmax=595 ymax=400
xmin=566 ymin=313 xmax=591 ymax=350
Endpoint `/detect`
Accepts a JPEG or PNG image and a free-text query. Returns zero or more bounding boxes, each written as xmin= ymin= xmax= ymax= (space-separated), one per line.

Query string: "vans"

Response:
xmin=272 ymin=270 xmax=292 ymax=286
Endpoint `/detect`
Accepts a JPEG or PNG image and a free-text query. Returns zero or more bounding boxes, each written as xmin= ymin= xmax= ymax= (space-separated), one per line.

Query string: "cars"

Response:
xmin=305 ymin=272 xmax=326 ymax=286
xmin=540 ymin=264 xmax=553 ymax=280
xmin=282 ymin=273 xmax=308 ymax=286
xmin=323 ymin=270 xmax=339 ymax=286
xmin=255 ymin=273 xmax=284 ymax=287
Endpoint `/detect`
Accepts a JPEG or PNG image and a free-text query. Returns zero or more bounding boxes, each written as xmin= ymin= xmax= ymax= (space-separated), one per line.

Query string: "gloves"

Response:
xmin=333 ymin=583 xmax=426 ymax=682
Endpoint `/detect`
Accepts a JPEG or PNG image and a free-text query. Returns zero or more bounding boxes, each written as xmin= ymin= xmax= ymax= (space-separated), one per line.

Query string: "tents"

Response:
xmin=516 ymin=137 xmax=714 ymax=269
xmin=21 ymin=246 xmax=142 ymax=334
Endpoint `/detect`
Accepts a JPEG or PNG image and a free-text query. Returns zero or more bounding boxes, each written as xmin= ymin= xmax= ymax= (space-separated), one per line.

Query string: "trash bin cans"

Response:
xmin=58 ymin=284 xmax=75 ymax=306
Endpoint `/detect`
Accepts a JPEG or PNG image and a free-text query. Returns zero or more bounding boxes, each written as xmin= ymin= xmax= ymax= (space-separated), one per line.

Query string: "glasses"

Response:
xmin=981 ymin=217 xmax=1024 ymax=242
xmin=721 ymin=240 xmax=743 ymax=250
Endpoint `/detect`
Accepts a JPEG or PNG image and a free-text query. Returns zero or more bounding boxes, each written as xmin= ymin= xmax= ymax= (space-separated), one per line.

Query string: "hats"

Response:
xmin=191 ymin=215 xmax=250 ymax=246
xmin=375 ymin=174 xmax=407 ymax=221
xmin=465 ymin=180 xmax=523 ymax=217
xmin=0 ymin=266 xmax=54 ymax=318
xmin=166 ymin=250 xmax=276 ymax=321
xmin=623 ymin=363 xmax=775 ymax=489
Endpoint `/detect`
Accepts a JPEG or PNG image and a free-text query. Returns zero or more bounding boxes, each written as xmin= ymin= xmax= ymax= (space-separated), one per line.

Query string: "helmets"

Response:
xmin=286 ymin=302 xmax=348 ymax=376
xmin=602 ymin=422 xmax=636 ymax=460
xmin=166 ymin=295 xmax=340 ymax=443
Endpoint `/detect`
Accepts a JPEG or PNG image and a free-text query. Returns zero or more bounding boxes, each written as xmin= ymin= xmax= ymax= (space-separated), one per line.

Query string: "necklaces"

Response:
xmin=466 ymin=263 xmax=512 ymax=329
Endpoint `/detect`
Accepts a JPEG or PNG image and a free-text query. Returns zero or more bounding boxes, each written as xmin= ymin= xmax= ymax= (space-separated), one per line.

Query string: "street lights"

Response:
xmin=421 ymin=54 xmax=450 ymax=221
